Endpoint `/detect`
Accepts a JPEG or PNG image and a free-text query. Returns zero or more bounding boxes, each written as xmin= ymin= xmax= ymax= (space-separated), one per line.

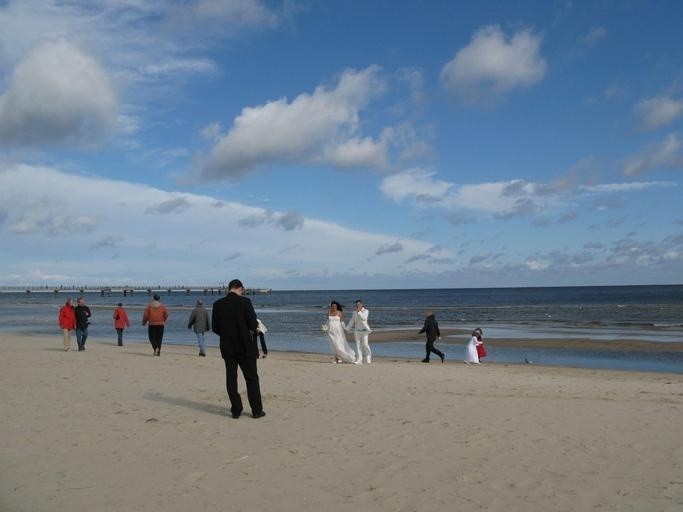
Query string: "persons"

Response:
xmin=142 ymin=294 xmax=167 ymax=356
xmin=113 ymin=303 xmax=129 ymax=346
xmin=475 ymin=328 xmax=487 ymax=362
xmin=211 ymin=279 xmax=265 ymax=418
xmin=187 ymin=298 xmax=209 ymax=356
xmin=346 ymin=299 xmax=371 ymax=364
xmin=74 ymin=298 xmax=90 ymax=351
xmin=59 ymin=298 xmax=76 ymax=350
xmin=321 ymin=300 xmax=356 ymax=363
xmin=417 ymin=310 xmax=444 ymax=363
xmin=255 ymin=319 xmax=268 ymax=358
xmin=464 ymin=331 xmax=483 ymax=365
